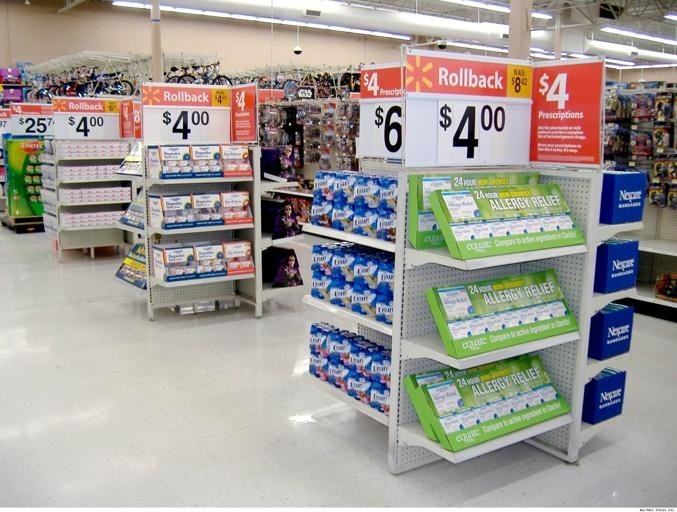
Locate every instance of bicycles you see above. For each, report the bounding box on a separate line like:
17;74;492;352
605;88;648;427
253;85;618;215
163;61;234;86
233;66;362;99
24;66;135;97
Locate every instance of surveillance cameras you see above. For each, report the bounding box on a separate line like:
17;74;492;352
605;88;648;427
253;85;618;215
437;39;447;50
293;45;303;56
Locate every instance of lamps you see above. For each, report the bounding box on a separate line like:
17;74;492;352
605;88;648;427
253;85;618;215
588;40;677;60
621;65;677;70
112;2;411;41
600;25;677;46
531;13;552;20
443;0;510;14
664;14;676;21
570;54;634;66
531;52;565;60
531;47;566;56
604;64;620;70
446;41;508;53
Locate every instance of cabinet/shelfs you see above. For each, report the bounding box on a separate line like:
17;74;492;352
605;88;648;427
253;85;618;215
0;133;53;234
601;87;677;309
37;140;143;264
258;103;360;226
299;158;644;476
112;141;304;321
